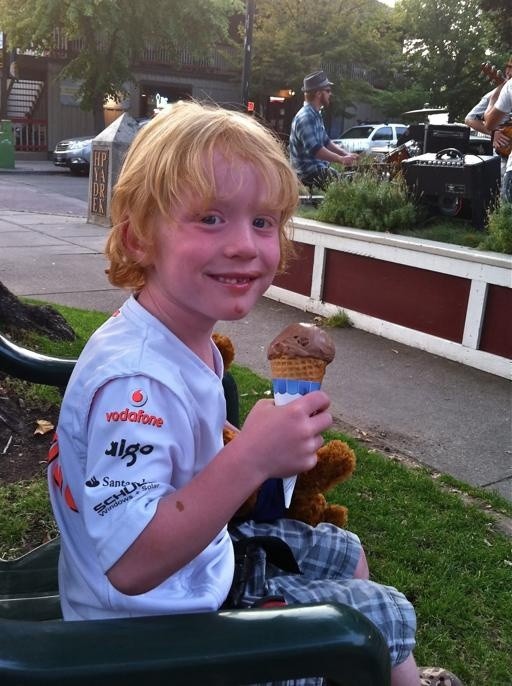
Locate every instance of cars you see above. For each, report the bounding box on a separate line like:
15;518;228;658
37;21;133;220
53;118;154;175
331;123;410;154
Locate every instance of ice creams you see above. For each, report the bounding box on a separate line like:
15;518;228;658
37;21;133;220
269;323;336;510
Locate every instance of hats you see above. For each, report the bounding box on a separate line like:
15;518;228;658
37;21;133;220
301;70;337;92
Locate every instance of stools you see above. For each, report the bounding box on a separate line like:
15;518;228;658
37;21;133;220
301;179;324;206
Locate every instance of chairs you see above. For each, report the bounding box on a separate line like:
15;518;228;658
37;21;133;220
0;332;392;685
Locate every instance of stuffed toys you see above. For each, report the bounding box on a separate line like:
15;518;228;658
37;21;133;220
210;333;357;530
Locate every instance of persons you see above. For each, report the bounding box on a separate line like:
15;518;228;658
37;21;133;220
464;64;512;186
46;98;461;686
485;76;512;205
290;70;357;193
